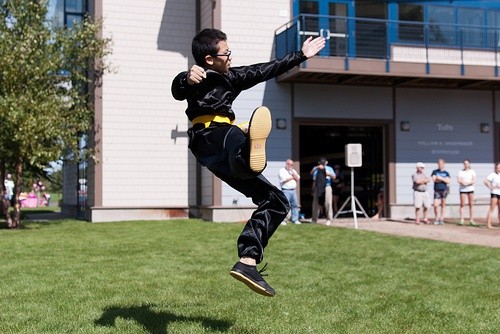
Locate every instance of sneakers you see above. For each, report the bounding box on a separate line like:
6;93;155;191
247;106;272;174
230;261;276;297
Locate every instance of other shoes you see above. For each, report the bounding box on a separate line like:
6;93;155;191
433;220;439;225
326;220;331;226
281;222;287;225
440;220;444;224
469;220;477;226
294;220;301;224
416;218;420;223
424;219;430;224
459;220;465;225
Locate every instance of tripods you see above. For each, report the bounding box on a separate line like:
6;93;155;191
333;166;374;230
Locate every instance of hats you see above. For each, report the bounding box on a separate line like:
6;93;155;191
416;162;424;167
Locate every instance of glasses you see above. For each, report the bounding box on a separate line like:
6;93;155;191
211;51;232;61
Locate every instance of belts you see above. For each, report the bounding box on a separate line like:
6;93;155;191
414;189;426;192
282;188;295;190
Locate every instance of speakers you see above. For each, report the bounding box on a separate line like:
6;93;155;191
345;144;363;167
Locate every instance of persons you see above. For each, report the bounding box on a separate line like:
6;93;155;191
331;164;343;214
0;173;51;230
278;159;301;225
457;160;476;226
411;162;431;225
311;157;337;226
483;162;500;228
171;28;326;296
430;159;451;226
